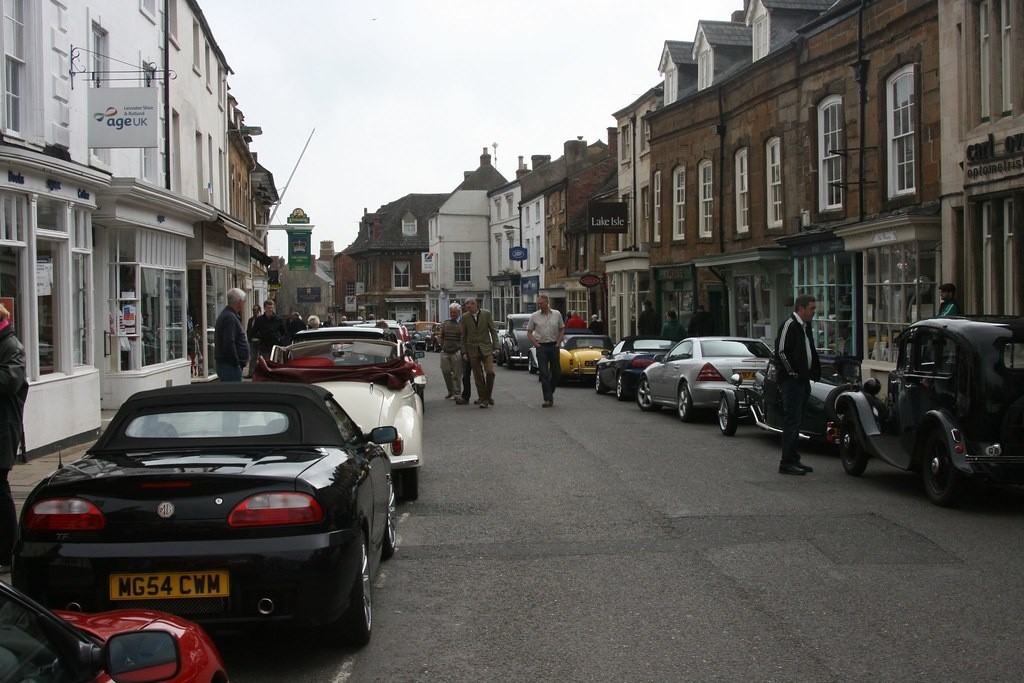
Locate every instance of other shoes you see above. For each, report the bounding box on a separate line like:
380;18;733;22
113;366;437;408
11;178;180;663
0;562;12;574
457;398;469;405
543;400;554;407
242;374;252;379
794;460;813;473
481;403;487;408
474;398;482;404
779;463;805;475
489;397;493;405
445;393;453;399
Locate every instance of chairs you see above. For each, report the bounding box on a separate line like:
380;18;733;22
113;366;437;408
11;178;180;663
139;422;177;438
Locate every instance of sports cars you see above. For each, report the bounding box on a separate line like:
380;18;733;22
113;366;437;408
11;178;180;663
10;380;402;648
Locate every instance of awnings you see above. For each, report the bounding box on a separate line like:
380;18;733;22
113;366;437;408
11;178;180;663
216;217;265;254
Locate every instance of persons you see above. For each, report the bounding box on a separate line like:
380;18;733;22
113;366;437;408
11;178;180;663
460;296;500;408
566;311;587;329
438;302;461;399
284;310;307;346
323;315;335;327
688;305;714;336
252;299;286;354
589;314;604;335
215;287;249;382
639;300;662;336
308;314;322;329
938;283;957;316
0;302;29;573
527;295;565;407
357;314;375;321
338;316;346;326
456;355;484;404
775;295;821;475
243;304;261;378
662;310;687;343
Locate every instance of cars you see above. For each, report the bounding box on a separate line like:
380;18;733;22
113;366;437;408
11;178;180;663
634;336;775;423
717;348;893;457
248;312;618;500
596;335;685;401
0;576;233;683
823;313;1024;507
